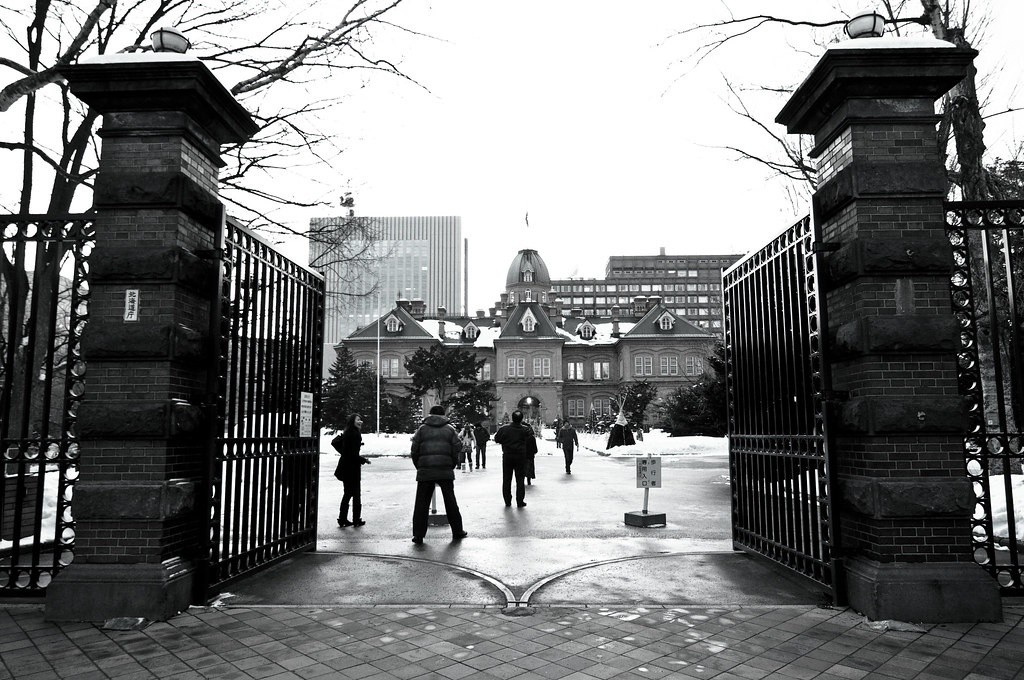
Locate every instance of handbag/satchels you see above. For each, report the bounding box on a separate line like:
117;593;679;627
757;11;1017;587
331;433;343;454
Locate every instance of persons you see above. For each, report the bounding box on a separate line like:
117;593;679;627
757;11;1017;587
411;405;467;543
494;410;542;507
334;414;371;527
556;414;579;474
458;423;490;472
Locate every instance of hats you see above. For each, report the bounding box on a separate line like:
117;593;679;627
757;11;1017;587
464;422;470;430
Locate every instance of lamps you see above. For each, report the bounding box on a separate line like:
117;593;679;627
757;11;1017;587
150;26;192;57
845;11;887;39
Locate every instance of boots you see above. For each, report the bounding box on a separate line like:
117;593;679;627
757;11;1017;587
469;462;473;472
461;463;466;473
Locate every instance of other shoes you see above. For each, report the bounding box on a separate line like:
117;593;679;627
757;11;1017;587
339;520;350;527
412;536;423;544
354;519;366;527
453;531;468;538
506;503;511;506
518;502;526;507
